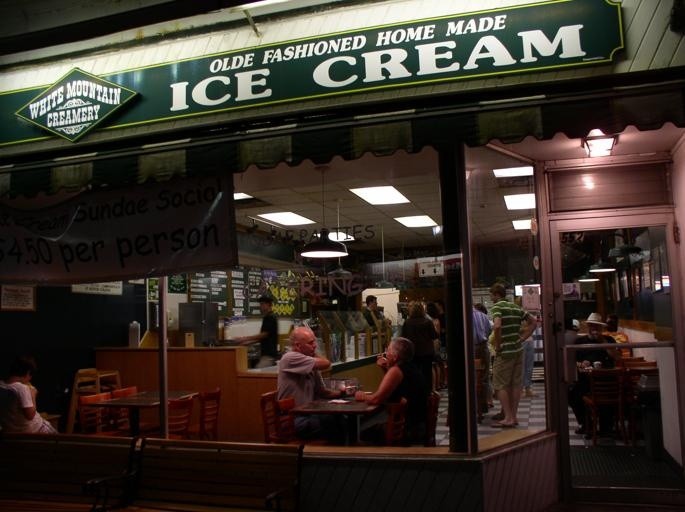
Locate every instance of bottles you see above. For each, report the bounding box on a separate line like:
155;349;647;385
128;321;140;347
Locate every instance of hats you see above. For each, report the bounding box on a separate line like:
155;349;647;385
572;320;580;330
581;313;608;328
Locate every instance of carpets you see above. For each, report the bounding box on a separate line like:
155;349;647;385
569;442;684;488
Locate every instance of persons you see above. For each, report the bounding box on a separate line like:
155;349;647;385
277;327;355;442
473;283;538;427
234;294;278;368
400;300;448;393
1;355;59;434
363;295;391;332
565;312;633;434
356;338;419;447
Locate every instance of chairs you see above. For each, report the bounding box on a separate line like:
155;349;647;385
576;356;663;458
65;367;441;449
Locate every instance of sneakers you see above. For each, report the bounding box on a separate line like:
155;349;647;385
526;388;539;397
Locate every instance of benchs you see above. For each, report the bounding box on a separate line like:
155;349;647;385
1;430;135;512
97;437;307;511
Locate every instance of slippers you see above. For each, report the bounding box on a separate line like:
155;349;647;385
491;412;518;428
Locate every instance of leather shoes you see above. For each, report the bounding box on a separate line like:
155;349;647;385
575;417;592;434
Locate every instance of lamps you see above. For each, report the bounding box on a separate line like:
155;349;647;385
298;166;349;259
579;127;620;159
327;199;353;280
373;220;396;289
